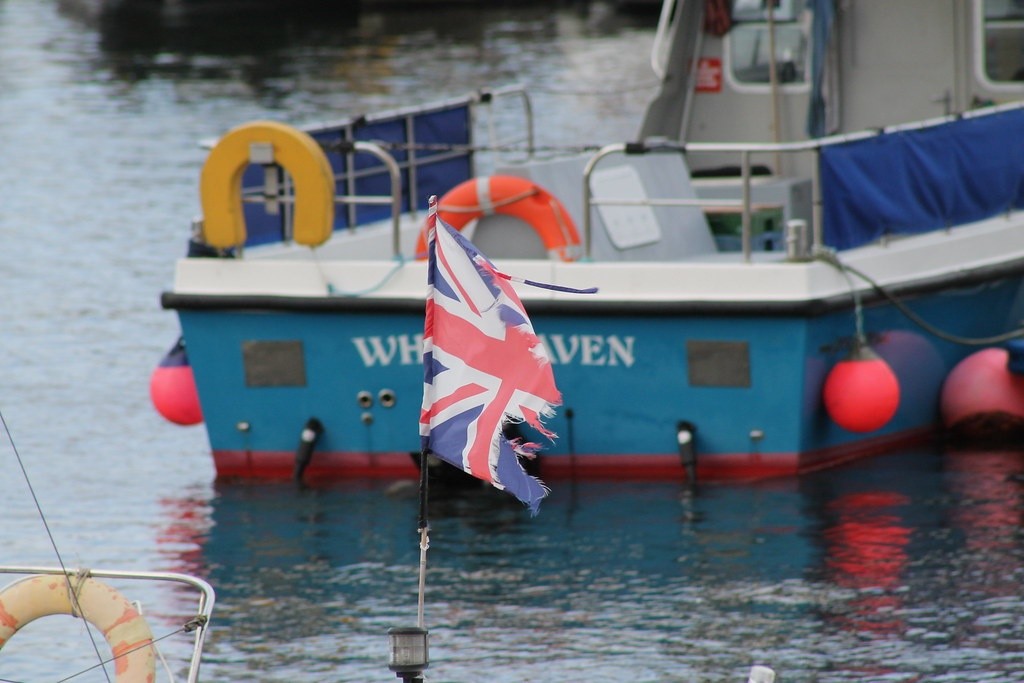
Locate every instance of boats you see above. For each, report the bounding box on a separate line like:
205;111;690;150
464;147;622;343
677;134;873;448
159;0;1024;484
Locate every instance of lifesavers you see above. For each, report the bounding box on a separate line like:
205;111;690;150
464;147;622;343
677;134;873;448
200;123;336;249
1;574;155;683
417;176;581;262
